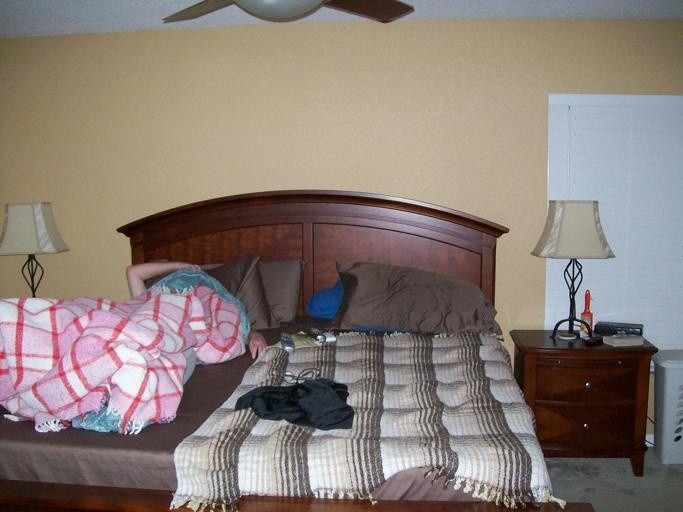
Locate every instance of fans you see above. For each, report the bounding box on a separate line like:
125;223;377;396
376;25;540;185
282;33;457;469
162;0;414;24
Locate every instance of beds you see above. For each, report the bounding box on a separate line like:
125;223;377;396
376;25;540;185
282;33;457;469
0;189;566;512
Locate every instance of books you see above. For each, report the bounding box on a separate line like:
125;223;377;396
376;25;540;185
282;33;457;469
594;321;643;336
602;334;644;347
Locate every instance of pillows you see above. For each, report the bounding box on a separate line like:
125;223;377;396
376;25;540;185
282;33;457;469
258;258;310;322
329;262;504;341
206;251;280;330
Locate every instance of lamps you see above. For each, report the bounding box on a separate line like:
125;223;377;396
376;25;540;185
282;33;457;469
233;0;322;22
531;200;616;337
0;203;70;298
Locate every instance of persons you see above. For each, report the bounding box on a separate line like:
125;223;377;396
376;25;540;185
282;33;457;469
127;261;268;360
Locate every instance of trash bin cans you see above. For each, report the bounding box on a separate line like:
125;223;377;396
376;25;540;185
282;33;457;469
650;350;683;465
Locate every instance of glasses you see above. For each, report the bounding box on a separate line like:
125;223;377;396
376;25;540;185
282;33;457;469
270;366;304;382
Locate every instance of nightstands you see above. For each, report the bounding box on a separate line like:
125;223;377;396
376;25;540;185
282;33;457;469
509;330;658;477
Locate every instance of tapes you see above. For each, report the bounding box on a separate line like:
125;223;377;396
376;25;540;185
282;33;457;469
557;332;578;340
